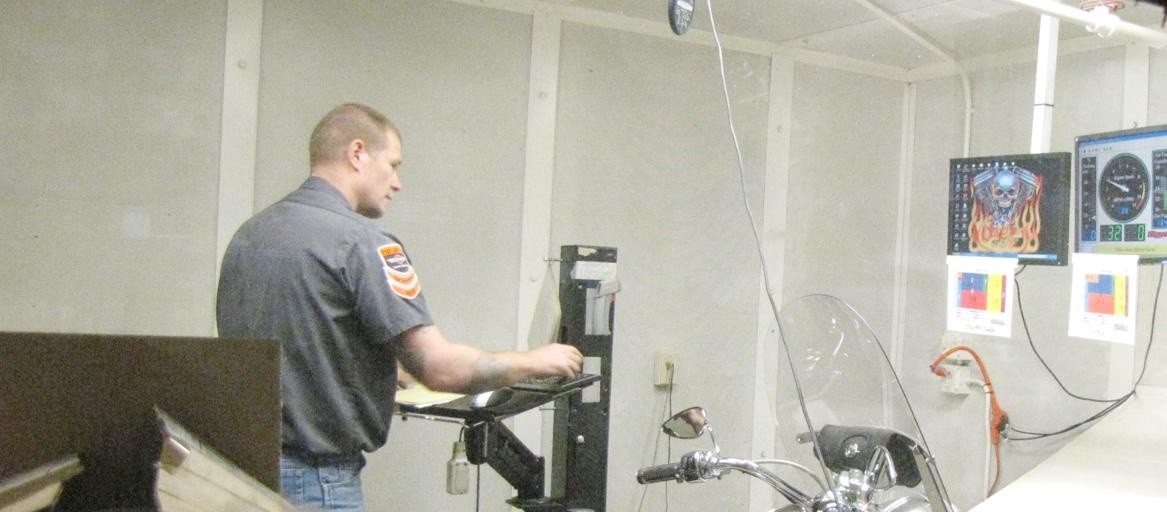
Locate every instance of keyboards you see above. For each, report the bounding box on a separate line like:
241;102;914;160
512;374;600;395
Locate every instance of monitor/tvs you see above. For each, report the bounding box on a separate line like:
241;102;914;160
945;151;1070;266
1075;127;1149;254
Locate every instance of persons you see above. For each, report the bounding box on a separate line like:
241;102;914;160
218;102;585;512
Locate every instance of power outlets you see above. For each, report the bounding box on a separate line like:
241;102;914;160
653;349;678;385
936;358;973;395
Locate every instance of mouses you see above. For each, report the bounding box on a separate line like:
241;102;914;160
469;385;510;408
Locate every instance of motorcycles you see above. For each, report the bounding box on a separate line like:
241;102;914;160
634;295;969;512
